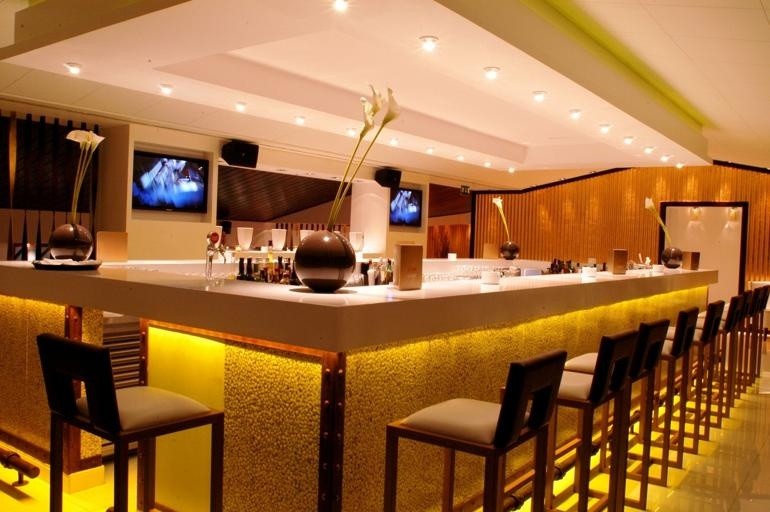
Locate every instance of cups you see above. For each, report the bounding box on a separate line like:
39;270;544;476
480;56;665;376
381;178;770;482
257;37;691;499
349;232;364;251
653;265;664;272
216;226;222;248
300;230;314;243
481;271;500;284
581;267;597;277
272;229;286;251
237;228;253;250
448;253;456;261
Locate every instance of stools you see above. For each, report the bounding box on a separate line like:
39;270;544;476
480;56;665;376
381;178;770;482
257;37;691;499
500;329;640;512
384;349;569;512
563;276;769;512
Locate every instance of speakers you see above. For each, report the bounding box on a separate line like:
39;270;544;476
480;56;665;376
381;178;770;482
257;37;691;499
375;168;401;188
222;139;259;168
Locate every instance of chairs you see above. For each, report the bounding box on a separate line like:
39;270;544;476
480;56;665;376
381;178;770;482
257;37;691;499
37;330;224;512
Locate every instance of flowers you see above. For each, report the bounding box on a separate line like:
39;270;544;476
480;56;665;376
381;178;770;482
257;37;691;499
491;197;512;242
326;85;402;232
643;197;675;247
64;128;106;226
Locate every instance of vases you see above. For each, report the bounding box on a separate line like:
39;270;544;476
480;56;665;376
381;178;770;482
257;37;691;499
500;241;520;260
660;246;682;270
49;221;95;262
291;227;356;295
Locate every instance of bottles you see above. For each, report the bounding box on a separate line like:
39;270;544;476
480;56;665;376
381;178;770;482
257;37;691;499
358;257;393;285
546;257;576;273
236;256;295;284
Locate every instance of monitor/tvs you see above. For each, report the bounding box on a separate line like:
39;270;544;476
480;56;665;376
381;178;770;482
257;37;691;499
389;187;422;229
132;146;210;216
